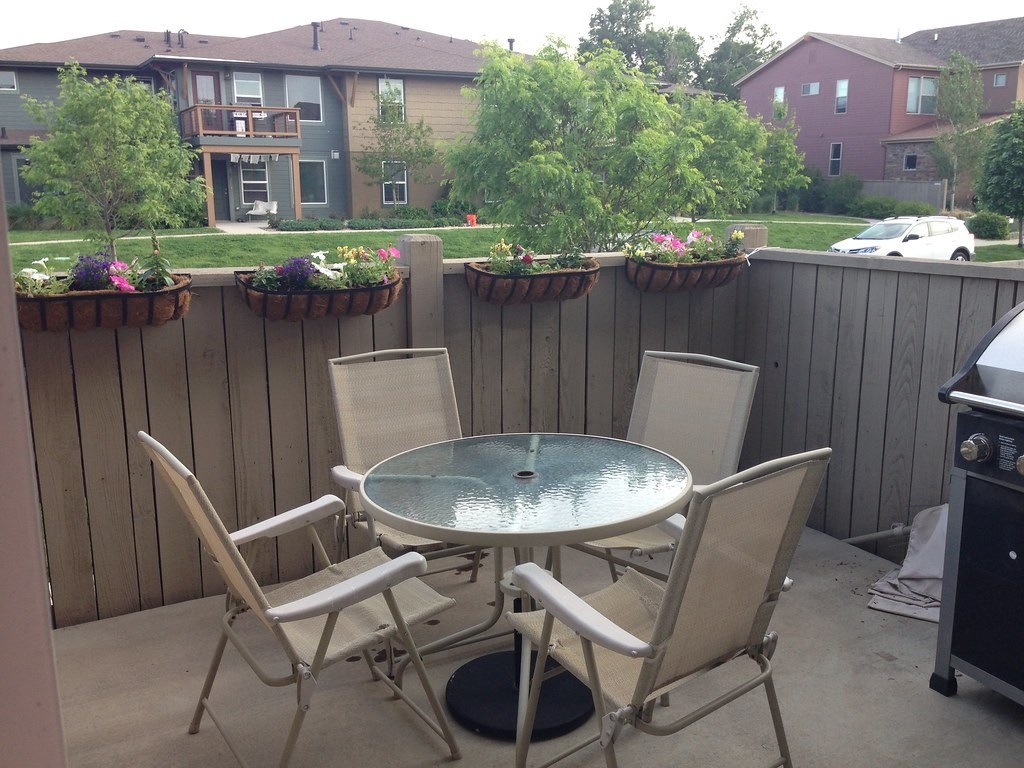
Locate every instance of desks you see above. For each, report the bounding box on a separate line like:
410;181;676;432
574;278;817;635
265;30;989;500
361;432;694;742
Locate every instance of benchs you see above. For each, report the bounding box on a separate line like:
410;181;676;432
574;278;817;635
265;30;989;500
245;199;278;222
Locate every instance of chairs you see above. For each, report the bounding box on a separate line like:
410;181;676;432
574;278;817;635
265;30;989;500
328;346;533;662
504;446;833;768
139;430;460;768
544;350;760;586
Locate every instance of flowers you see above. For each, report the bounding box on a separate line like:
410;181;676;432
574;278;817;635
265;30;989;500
623;226;745;265
488;229;587;274
12;226;174;296
250;243;401;293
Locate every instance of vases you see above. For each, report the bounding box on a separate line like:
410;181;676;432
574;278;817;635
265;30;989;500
625;251;746;293
14;275;190;332
235;270;401;323
463;256;600;304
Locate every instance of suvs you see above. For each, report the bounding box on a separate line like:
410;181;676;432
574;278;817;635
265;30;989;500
827;214;975;262
